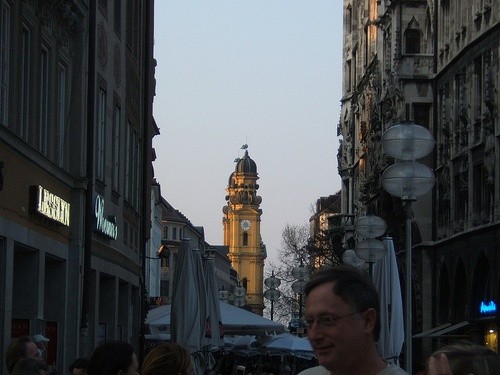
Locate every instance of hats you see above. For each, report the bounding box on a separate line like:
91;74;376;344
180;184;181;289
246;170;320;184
32;335;50;345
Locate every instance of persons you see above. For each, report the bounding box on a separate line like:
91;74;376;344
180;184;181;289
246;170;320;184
86;339;140;375
239;359;309;375
426;341;500;375
6;334;60;375
137;342;194;375
69;358;89;375
296;263;409;375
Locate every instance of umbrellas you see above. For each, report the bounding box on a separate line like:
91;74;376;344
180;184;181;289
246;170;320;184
372;233;404;368
169;238;225;375
220;332;317;360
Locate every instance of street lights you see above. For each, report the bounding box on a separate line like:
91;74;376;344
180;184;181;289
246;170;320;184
379;103;435;375
263;270;281;321
291;259;311;338
353;201;388;279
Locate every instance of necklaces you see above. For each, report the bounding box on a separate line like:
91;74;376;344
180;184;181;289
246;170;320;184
381;358;388;368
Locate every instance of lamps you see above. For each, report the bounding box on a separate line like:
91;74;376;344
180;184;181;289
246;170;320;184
141;245;170;261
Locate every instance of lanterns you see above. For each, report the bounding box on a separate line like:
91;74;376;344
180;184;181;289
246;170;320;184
264;289;281;301
380;161;436;201
292;265;310;281
355;214;387;239
265;276;281;289
379;120;436;162
354;238;387;263
341;249;366;267
234;286;246;296
291;279;307;295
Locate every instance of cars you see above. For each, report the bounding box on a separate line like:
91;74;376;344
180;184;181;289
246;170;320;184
288;318;307;334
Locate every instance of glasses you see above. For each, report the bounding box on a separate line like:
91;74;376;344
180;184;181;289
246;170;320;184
299;309;362;330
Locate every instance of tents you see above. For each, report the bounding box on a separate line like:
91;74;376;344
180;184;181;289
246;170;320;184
144;303;284;333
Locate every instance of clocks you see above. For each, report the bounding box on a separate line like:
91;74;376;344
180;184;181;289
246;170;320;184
241;219;252;230
